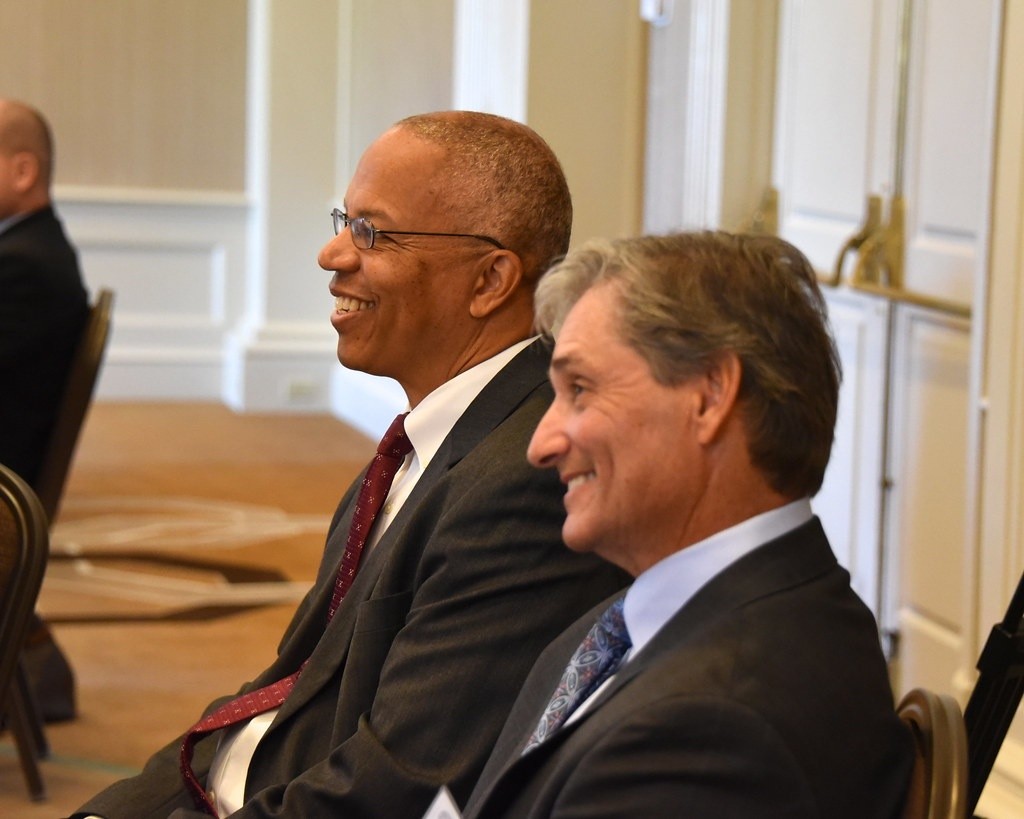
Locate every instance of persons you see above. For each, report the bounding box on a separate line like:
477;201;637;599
60;111;624;818
0;95;92;501
442;229;918;818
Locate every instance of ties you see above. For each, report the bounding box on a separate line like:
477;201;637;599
179;408;415;819
519;590;632;754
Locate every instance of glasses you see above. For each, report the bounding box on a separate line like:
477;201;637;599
330;207;506;252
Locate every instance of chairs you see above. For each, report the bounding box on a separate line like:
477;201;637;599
2;281;118;802
